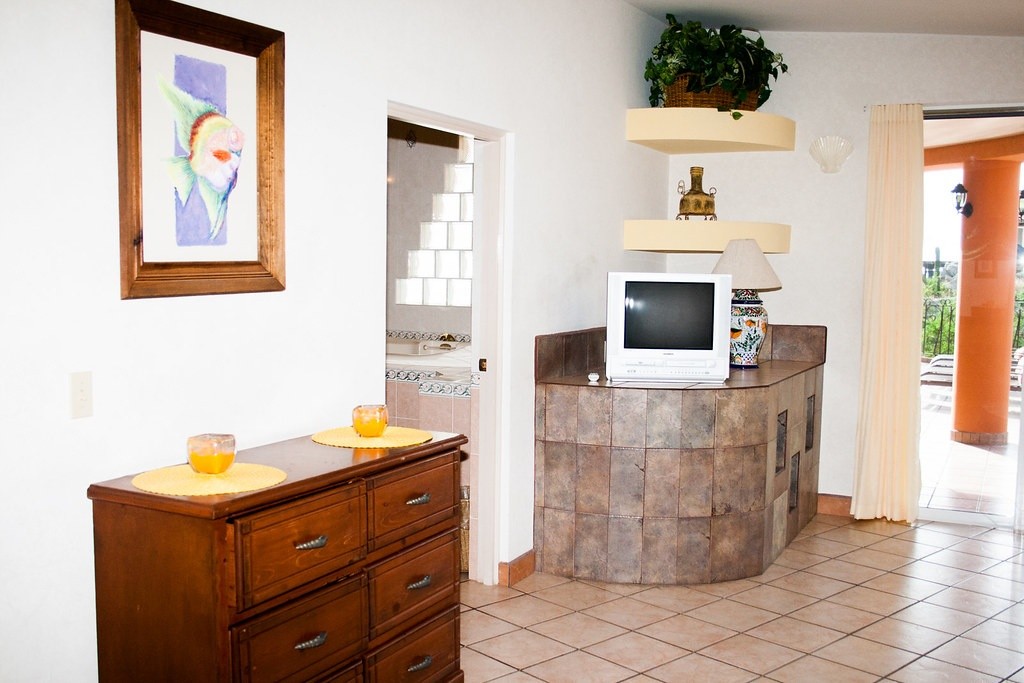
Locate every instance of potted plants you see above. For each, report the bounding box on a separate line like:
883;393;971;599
643;14;789;120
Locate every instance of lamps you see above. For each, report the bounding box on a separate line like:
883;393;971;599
1018;189;1024;229
951;184;973;218
713;239;783;370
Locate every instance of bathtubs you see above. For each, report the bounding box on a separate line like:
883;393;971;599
387;339;471;486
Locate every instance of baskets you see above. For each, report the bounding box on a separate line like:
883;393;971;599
662;72;758;112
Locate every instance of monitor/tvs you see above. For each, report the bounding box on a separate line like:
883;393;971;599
606;272;732;387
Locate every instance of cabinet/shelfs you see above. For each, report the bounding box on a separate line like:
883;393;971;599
626;106;796;254
87;425;465;683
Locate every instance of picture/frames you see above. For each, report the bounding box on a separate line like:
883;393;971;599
116;0;286;299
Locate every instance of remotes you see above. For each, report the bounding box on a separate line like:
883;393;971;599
588;373;599;381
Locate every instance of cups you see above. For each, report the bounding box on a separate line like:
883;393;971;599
188;434;236;474
354;405;389;438
